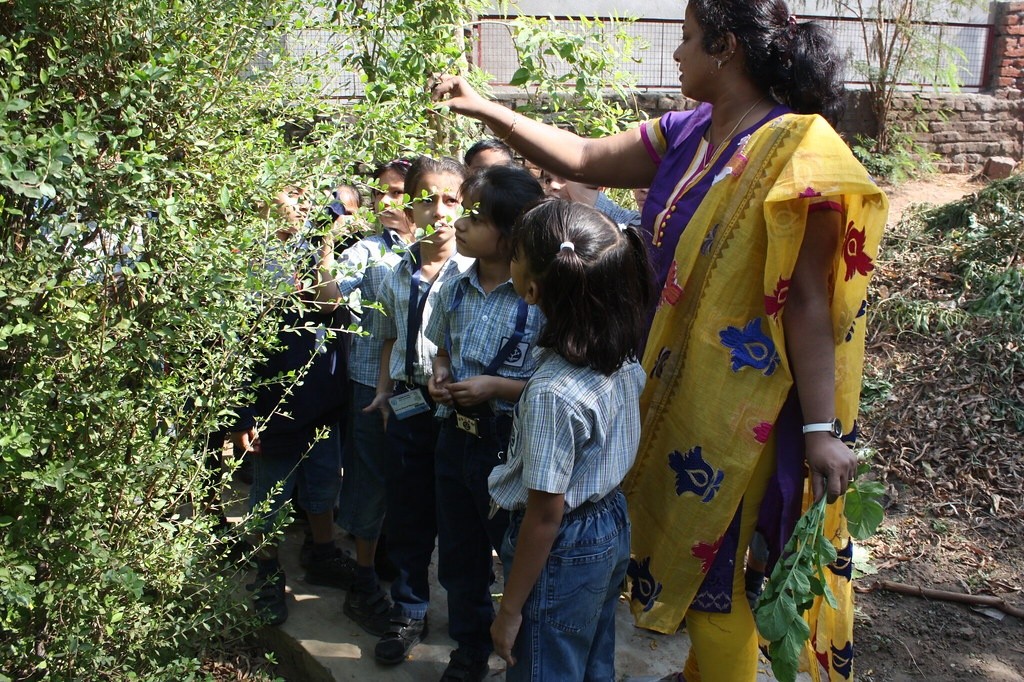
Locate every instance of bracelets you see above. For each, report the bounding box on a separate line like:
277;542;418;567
494;111;516;141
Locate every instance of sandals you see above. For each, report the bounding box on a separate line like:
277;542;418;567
375;614;428;664
343;582;396;635
440;648;489;682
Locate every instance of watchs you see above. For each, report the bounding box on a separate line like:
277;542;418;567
803;418;843;438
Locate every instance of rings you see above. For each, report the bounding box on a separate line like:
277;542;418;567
849;477;855;482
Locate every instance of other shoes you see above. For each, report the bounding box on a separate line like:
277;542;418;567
207;524;254;567
235;448;255;486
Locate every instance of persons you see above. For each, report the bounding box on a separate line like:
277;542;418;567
14;137;655;682
488;199;656;682
427;1;889;682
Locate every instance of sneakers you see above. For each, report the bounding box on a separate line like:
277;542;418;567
246;565;289;626
303;541;357;588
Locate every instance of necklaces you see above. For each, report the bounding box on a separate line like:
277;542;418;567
703;97;762;170
